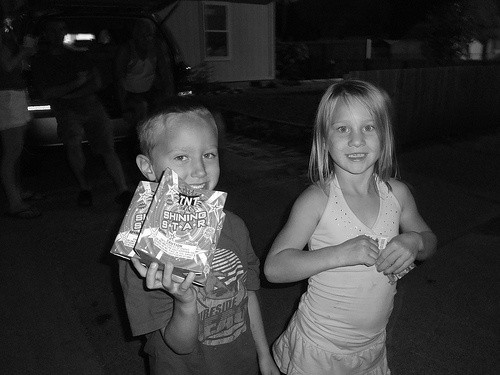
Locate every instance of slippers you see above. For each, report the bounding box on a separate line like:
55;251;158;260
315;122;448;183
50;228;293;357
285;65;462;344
7;189;46;218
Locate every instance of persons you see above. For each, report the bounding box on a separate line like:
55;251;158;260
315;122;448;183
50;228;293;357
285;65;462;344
117;17;170;123
0;18;47;218
367;40;403;70
262;78;437;375
33;20;134;207
108;98;282;375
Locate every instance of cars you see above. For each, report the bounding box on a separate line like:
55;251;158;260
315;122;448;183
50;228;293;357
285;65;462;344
18;0;195;169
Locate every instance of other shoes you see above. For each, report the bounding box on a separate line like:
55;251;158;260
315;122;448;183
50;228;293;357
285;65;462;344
80;189;93;207
119;190;132;201
